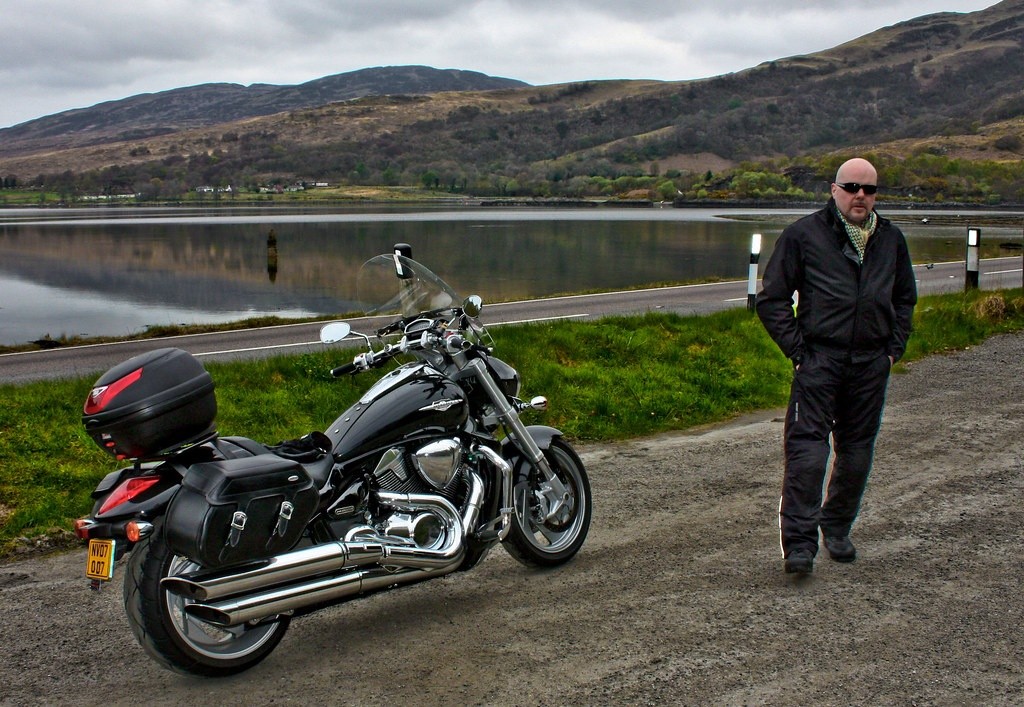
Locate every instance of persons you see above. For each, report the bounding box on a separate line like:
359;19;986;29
754;157;918;574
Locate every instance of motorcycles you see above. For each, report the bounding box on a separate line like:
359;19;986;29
75;252;590;679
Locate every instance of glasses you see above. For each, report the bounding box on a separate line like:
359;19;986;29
835;182;879;195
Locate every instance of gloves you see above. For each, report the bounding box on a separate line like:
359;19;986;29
262;431;333;463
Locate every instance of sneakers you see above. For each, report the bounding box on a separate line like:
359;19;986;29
822;532;857;563
785;549;815;574
